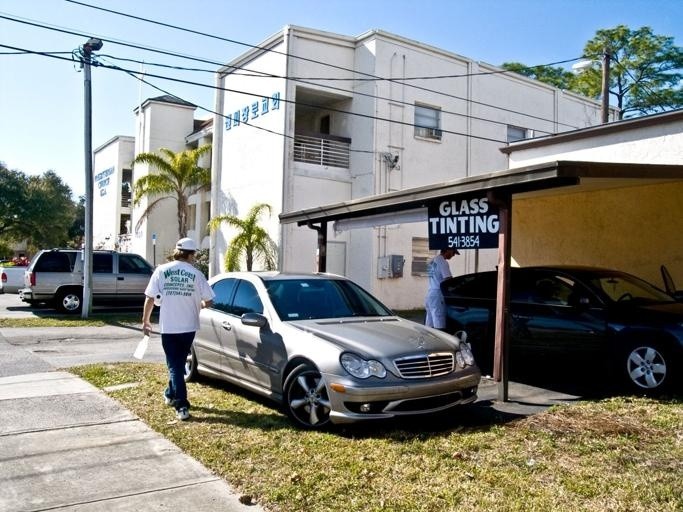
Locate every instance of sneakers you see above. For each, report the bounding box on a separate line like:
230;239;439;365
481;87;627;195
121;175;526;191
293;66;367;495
163;391;174;406
176;406;190;420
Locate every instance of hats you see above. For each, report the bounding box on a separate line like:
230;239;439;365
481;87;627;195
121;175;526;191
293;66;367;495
175;237;201;252
454;248;460;256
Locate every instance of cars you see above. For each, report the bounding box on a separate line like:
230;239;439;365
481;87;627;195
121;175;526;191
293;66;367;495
18;248;156;315
0;267;24;293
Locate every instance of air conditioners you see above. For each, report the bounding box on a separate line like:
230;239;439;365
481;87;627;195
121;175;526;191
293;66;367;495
428;127;443;138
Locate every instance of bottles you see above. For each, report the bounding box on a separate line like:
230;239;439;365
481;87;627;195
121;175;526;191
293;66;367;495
132;334;151;361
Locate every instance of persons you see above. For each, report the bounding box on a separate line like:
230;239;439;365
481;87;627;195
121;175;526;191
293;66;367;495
141;237;217;421
423;247;461;331
